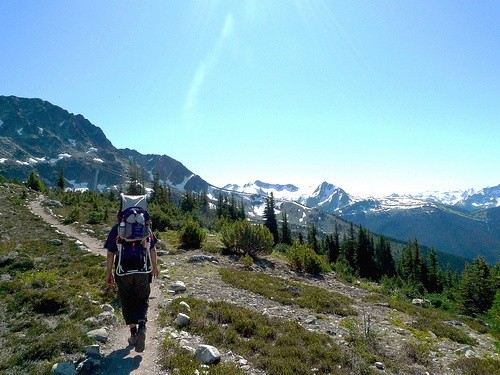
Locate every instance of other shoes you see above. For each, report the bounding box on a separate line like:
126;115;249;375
127;329;147;352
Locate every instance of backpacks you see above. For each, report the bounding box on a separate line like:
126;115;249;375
114;192;154;276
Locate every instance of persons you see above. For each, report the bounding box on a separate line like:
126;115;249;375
103;210;160;353
